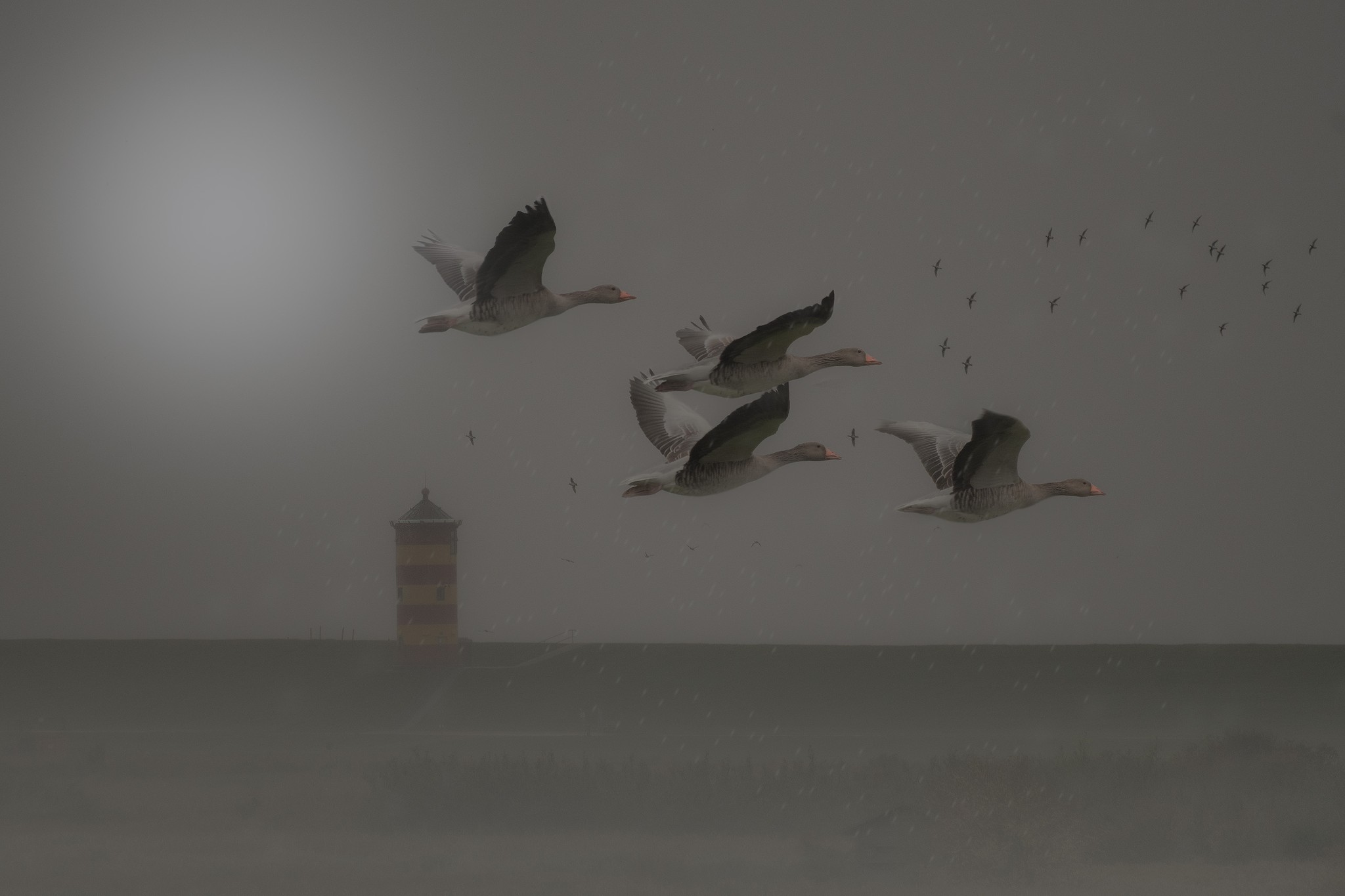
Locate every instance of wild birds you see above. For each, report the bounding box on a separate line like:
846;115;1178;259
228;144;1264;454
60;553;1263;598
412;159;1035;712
847;428;859;446
967;292;977;309
1262;279;1272;296
961;355;972;373
1208;236;1319;277
1179;284;1191;298
1219;322;1229;337
939;337;950;357
415;194;637;336
644;289;883;397
932;259;942;277
1144;210;1153;230
1045;227;1054;248
1191;215;1202;233
1079;228;1088;246
874;407;1107;521
1293;304;1302;322
462;429;766;561
620;368;842;496
1049;297;1061;314
